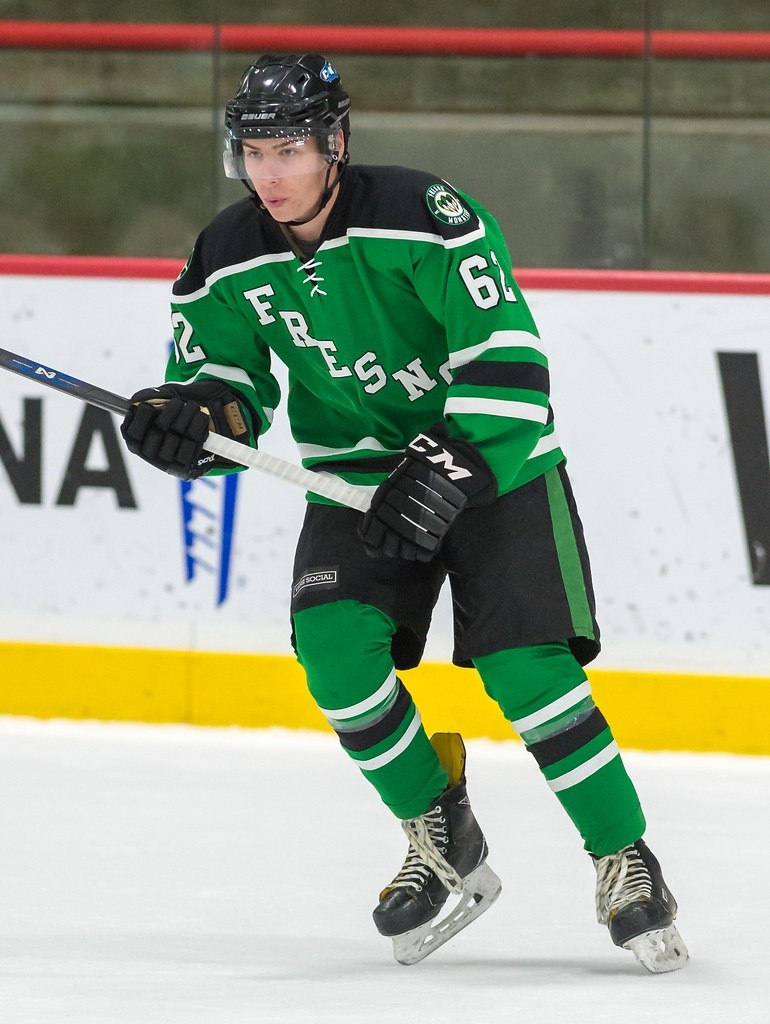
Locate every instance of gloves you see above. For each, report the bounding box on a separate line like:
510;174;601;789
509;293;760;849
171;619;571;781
120;384;249;480
359;424;497;567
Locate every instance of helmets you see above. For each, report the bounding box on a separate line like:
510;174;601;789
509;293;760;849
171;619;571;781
227;55;352;159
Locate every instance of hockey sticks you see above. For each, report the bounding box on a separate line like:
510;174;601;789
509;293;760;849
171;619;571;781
0;324;385;521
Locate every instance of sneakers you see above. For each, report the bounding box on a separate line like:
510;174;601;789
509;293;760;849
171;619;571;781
584;838;690;975
371;732;501;967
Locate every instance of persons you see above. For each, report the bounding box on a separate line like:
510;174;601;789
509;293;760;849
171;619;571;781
119;48;689;973
